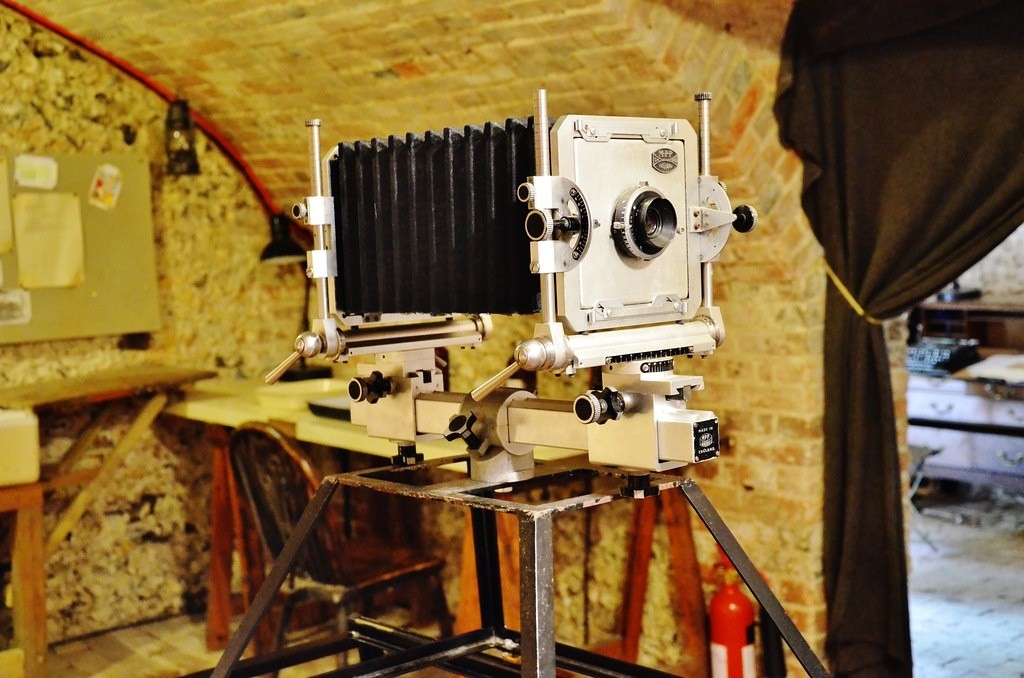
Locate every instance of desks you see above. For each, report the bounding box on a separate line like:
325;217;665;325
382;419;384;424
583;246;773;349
131;373;587;651
0;364;216;677
0;483;45;677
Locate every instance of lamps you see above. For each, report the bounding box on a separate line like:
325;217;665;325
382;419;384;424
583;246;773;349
161;100;203;175
257;213;332;383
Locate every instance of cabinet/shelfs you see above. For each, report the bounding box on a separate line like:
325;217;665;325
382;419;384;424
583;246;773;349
905;302;1024;494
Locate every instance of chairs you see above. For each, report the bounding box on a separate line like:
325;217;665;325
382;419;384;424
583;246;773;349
229;422;454;676
909;443;942;550
